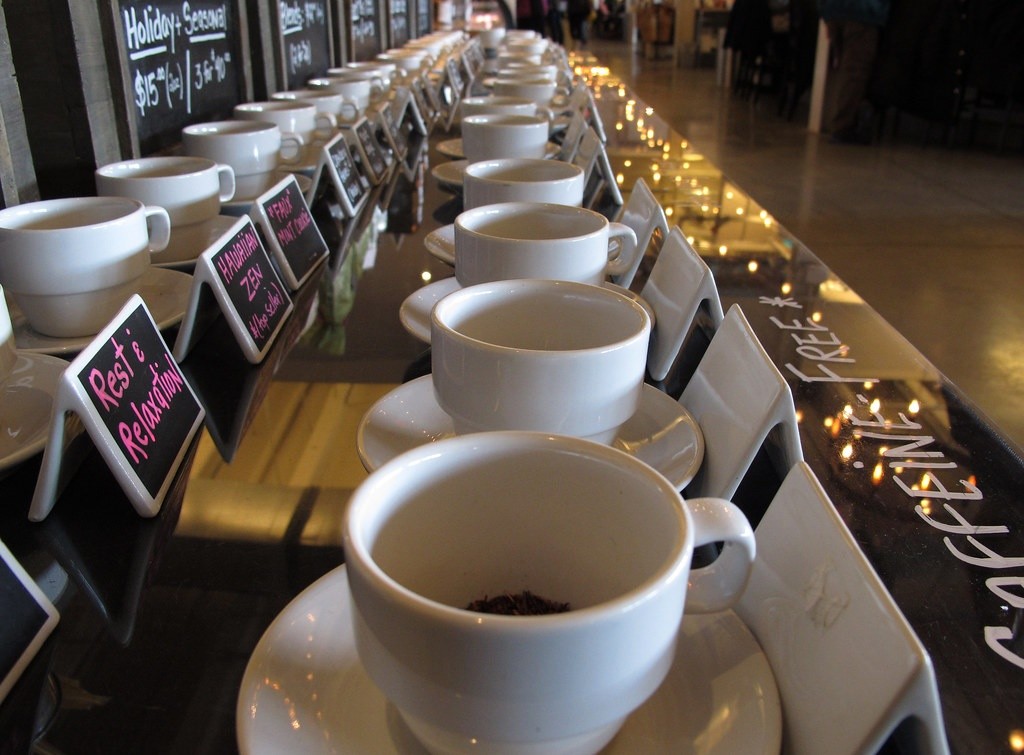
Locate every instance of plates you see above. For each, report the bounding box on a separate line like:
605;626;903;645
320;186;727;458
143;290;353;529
354;374;705;507
398;274;656;355
144;215;242;270
0;351;80;471
2;266;193;355
281;136;337;173
428;162;492;186
232;560;790;755
434;136;564;160
218;170;313;212
424;219;621;266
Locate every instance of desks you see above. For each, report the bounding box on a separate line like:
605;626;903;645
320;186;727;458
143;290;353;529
0;25;1024;755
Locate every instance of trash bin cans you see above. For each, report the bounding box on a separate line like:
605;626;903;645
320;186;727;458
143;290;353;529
717;27;741;89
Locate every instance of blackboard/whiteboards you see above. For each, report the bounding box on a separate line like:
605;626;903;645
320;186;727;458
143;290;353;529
110;0;254;161
389;0;432;49
277;0;334;90
349;0;386;62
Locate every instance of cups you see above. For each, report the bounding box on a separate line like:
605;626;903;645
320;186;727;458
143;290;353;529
343;25;756;755
0;23;476;474
